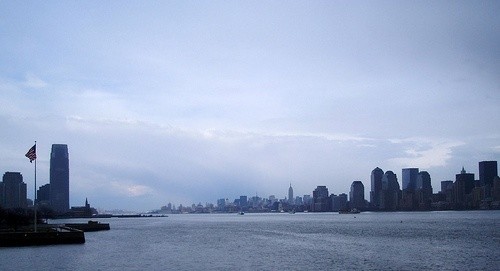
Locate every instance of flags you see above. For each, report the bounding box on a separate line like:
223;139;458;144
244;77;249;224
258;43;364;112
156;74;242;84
25;144;37;163
